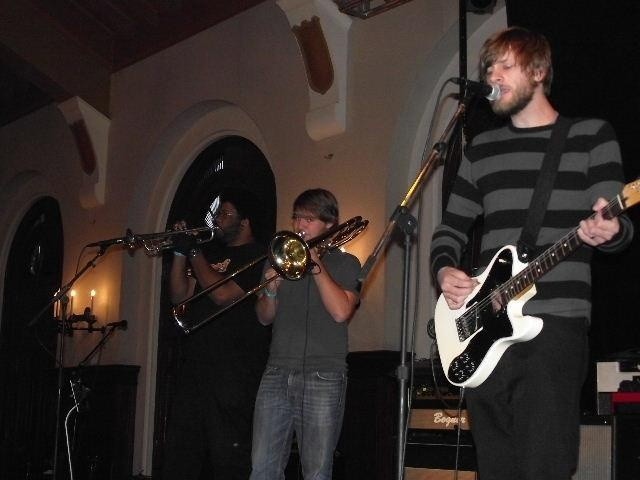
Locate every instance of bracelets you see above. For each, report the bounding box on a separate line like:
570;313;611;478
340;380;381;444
263;286;277;300
170;248;185;256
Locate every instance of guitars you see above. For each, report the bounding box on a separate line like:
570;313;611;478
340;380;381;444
433;178;639;391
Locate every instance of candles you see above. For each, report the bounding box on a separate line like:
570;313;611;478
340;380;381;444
90;296;94;314
70;296;73;317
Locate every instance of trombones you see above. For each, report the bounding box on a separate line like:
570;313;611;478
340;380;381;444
170;216;370;334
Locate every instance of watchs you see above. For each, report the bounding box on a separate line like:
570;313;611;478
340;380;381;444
190;249;200;256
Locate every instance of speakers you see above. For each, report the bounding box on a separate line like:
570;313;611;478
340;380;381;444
571;414;616;480
402;358;479;480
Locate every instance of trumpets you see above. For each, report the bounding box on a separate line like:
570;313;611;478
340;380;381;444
123;224;223;258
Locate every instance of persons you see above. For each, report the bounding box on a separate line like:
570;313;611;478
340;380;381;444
247;188;362;480
429;25;634;480
166;199;262;480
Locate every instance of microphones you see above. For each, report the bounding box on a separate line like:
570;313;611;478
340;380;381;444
107;320;128;327
451;76;503;102
87;236;131;247
